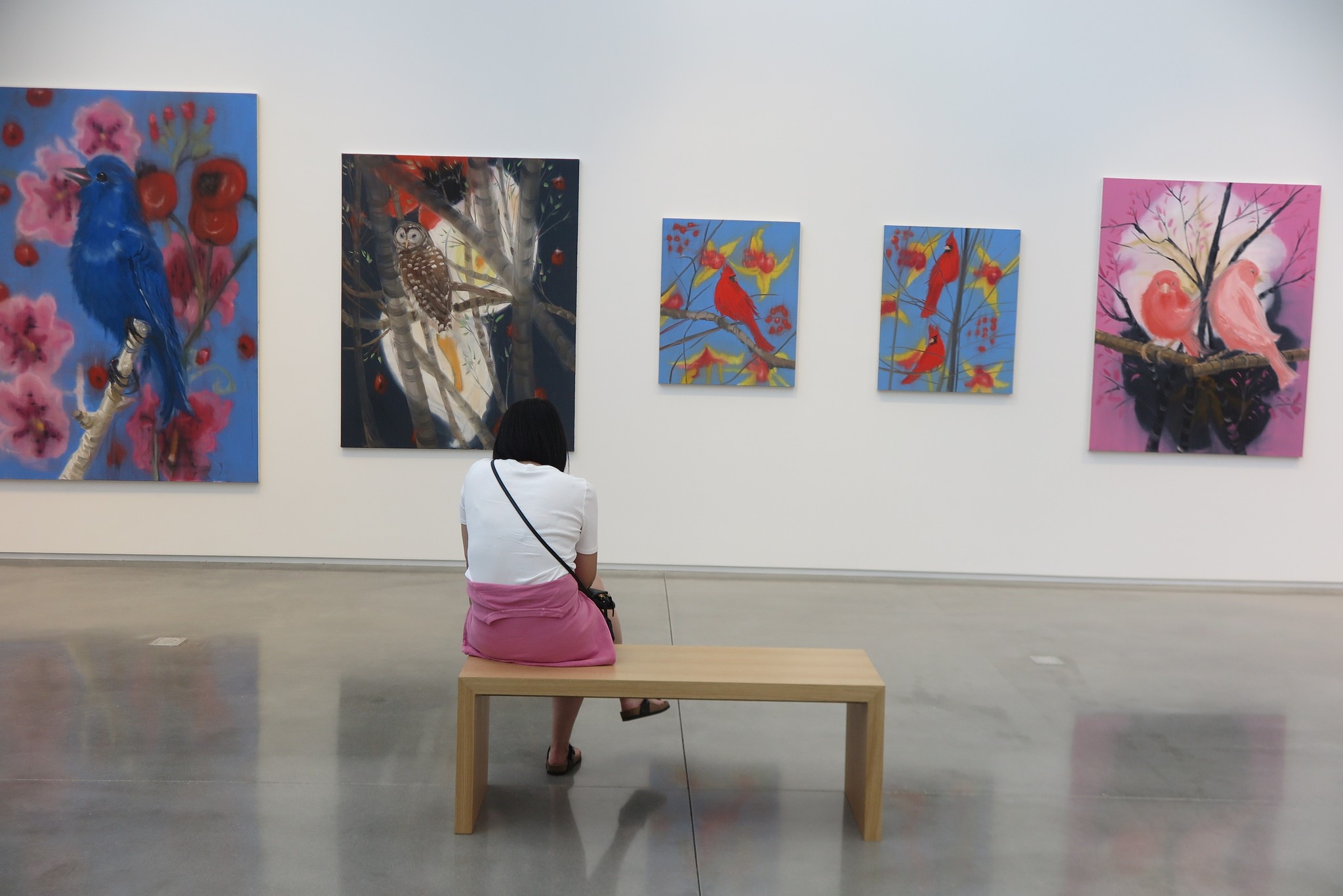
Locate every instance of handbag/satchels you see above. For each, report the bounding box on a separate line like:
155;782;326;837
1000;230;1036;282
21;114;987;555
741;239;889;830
588;586;616;642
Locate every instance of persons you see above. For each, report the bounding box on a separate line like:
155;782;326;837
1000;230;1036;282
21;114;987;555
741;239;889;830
458;398;671;775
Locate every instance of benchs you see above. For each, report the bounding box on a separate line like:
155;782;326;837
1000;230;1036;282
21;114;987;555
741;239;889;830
454;644;884;841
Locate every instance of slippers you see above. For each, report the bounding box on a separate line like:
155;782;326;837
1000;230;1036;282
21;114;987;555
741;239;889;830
620;697;670;721
545;742;582;775
